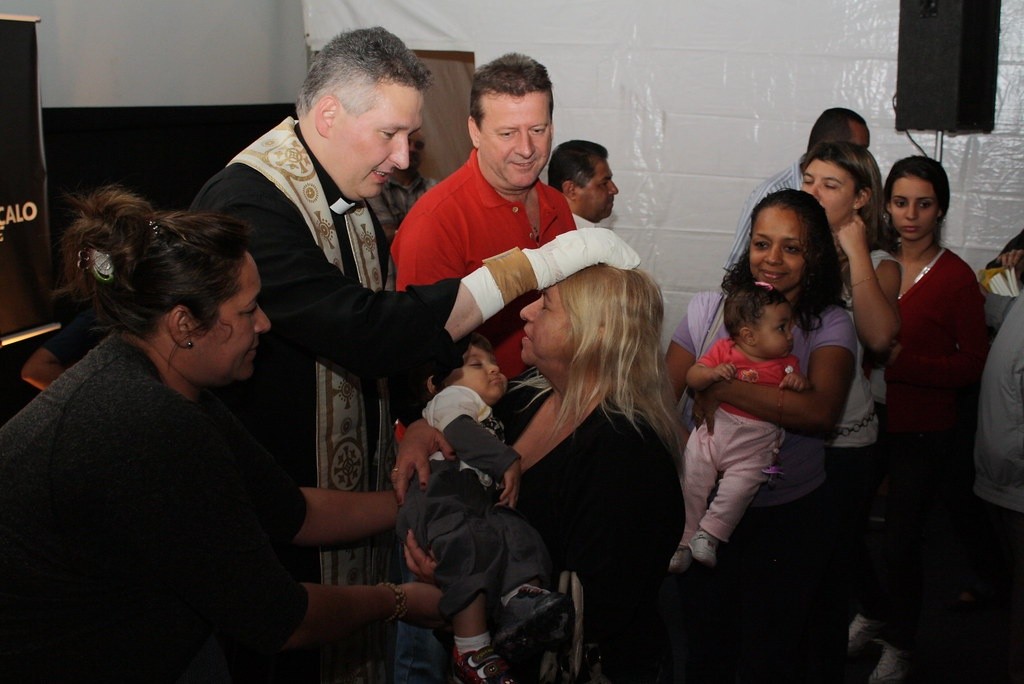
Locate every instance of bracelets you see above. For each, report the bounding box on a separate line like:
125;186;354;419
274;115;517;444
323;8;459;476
377;581;408;623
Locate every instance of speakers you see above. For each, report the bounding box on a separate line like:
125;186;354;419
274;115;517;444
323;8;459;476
895;0;1001;136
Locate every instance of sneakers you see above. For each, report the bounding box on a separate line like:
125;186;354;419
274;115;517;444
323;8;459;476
863;638;907;684
667;545;693;573
451;645;517;684
847;613;891;657
493;586;566;644
688;531;717;565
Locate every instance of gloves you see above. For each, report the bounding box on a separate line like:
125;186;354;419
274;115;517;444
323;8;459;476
482;227;641;308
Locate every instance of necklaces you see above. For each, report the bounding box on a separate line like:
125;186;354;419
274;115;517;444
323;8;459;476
530;222;540;242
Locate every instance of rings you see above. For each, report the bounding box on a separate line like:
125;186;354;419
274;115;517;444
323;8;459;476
392;467;400;472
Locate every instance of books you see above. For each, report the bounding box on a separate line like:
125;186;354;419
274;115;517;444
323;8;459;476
987;267;1019;297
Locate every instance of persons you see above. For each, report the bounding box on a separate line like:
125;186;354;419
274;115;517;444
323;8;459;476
960;225;1024;684
385;263;686;680
395;55;639;379
0;186;458;684
182;26;640;684
667;110;979;678
